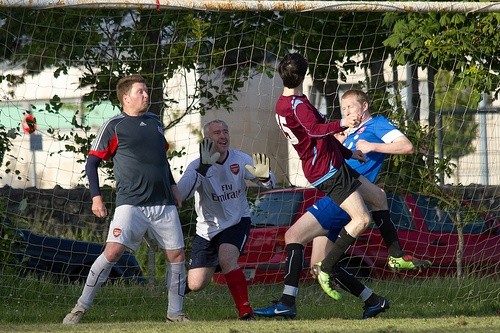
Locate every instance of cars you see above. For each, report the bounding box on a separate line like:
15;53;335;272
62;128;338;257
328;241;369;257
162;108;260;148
210;185;500;285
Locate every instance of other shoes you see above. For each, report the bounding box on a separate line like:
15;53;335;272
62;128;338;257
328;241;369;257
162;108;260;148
239;312;256;320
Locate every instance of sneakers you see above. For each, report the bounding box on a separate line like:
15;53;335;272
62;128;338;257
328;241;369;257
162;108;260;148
388;255;432;270
252;300;297;320
63;304;87;324
310;260;342;301
166;313;190;322
361;296;389;320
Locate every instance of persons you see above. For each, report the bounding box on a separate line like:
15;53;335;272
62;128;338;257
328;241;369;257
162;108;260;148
250;90;413;318
62;74;189;325
176;120;275;322
275;53;431;302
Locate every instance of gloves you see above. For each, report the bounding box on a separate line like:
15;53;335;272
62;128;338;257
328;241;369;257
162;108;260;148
195;138;220;177
245;153;271;183
